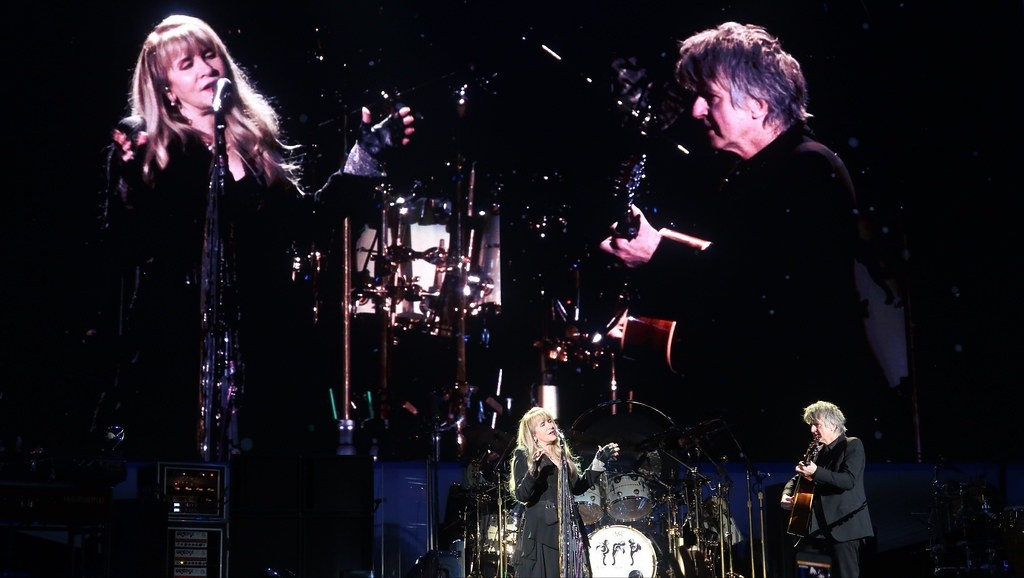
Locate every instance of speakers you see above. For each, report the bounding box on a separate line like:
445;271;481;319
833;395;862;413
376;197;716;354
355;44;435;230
228;453;375;578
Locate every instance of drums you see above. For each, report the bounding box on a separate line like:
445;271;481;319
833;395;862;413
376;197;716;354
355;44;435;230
349;207;503;329
477;513;520;562
570;482;604;527
588;525;657;578
604;472;653;523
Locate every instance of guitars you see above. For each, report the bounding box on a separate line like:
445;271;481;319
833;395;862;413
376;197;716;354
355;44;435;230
785;433;823;537
615;150;687;381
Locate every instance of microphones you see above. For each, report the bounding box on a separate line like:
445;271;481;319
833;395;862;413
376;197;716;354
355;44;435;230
560;441;565;460
211;77;232;111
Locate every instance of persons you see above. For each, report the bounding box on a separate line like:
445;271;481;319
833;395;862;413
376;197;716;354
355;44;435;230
509;406;620;578
108;13;415;477
599;22;865;532
781;401;874;577
